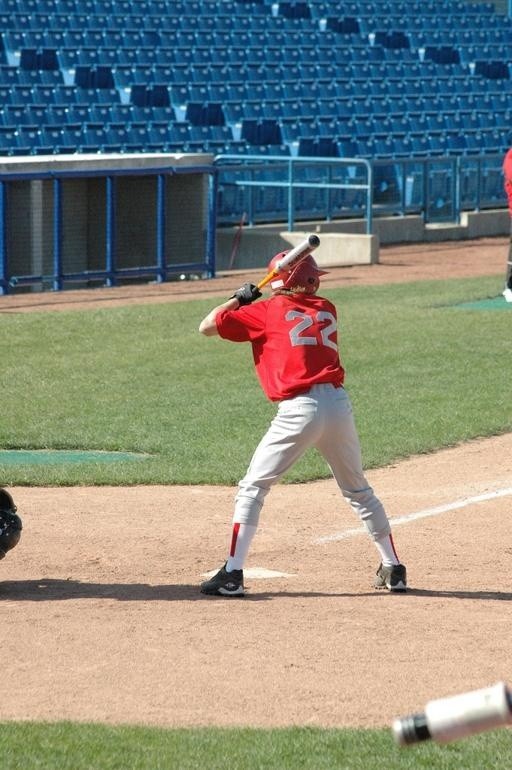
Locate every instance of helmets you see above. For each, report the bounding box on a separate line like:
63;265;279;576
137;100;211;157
267;248;330;294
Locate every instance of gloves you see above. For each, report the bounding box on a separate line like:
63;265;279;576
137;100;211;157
233;285;263;306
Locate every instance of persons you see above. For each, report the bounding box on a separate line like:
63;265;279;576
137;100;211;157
198;248;411;599
500;148;512;304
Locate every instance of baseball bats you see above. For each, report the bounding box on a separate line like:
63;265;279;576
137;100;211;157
252;236;320;292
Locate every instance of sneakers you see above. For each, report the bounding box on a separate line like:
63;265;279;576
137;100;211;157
368;562;409;593
198;564;247;596
